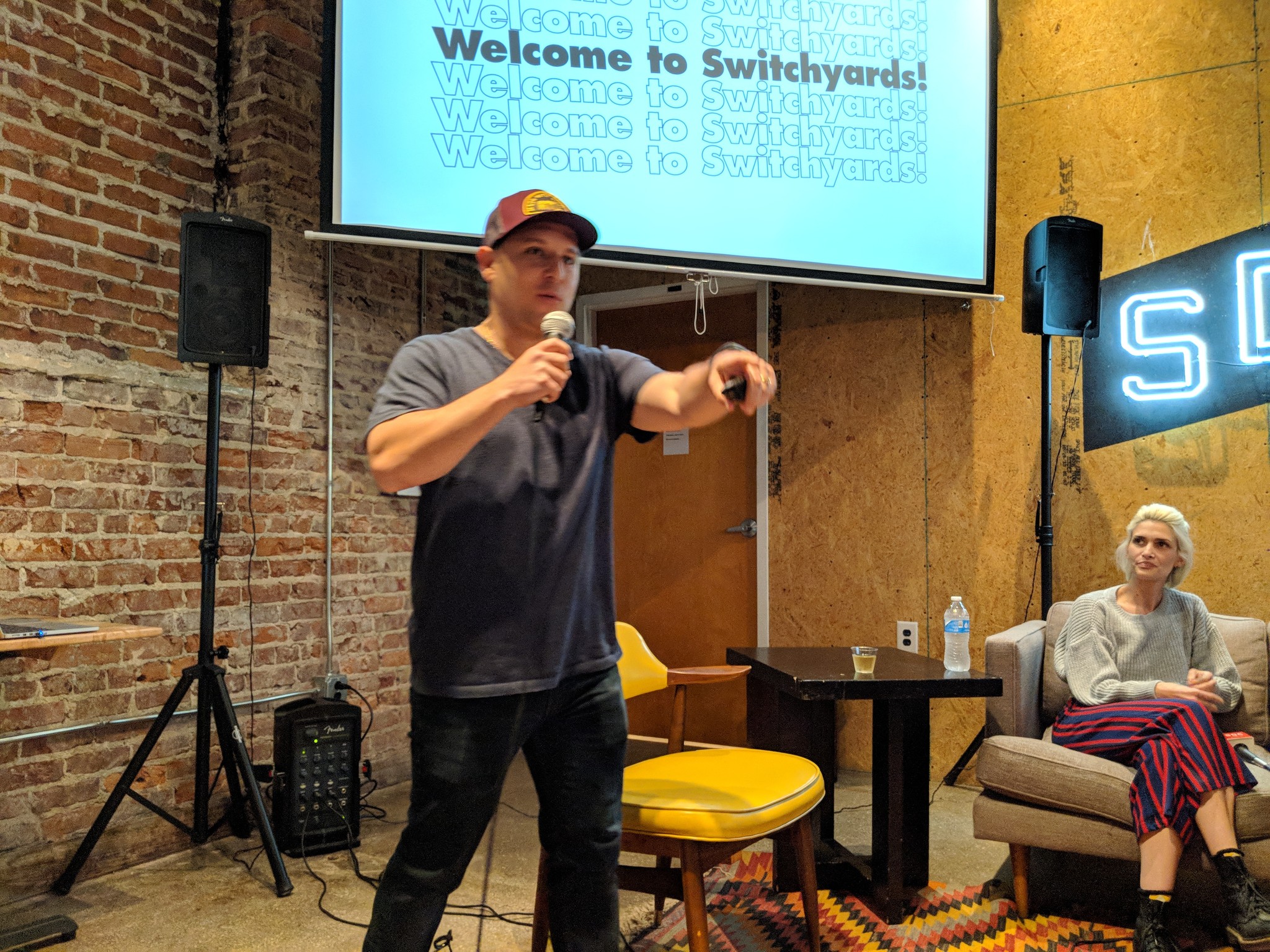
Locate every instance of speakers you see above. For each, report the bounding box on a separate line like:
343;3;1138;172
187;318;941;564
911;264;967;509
176;212;271;368
1021;216;1104;341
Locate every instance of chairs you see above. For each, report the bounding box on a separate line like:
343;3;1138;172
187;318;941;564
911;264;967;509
533;618;824;952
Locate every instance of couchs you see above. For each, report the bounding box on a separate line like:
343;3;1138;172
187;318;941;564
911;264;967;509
972;600;1270;922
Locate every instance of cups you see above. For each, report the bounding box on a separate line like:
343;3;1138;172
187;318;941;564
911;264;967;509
850;646;878;674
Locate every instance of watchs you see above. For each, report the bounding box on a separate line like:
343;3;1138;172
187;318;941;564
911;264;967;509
708;341;750;364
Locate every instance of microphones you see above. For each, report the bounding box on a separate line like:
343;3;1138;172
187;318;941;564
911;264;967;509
1223;731;1270;772
534;311;575;422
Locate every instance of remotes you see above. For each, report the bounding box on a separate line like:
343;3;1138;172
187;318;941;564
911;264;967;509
721;376;746;401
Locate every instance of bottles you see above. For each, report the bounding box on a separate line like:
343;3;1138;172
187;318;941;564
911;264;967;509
943;595;971;672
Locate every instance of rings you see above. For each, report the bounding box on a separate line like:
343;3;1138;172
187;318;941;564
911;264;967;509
760;375;772;385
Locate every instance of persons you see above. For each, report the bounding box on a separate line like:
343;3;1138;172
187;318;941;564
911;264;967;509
362;186;777;952
1052;503;1270;952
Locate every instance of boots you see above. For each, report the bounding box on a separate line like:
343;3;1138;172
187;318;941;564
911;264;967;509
1212;856;1270;952
1133;896;1172;952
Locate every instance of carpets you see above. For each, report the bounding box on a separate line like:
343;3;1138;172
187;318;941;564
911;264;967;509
618;850;1238;952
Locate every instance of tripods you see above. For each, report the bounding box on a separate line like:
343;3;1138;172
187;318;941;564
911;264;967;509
50;361;294;898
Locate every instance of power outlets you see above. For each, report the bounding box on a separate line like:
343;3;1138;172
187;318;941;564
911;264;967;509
896;620;918;654
314;673;349;701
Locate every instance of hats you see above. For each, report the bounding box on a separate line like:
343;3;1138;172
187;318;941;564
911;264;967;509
483;189;597;251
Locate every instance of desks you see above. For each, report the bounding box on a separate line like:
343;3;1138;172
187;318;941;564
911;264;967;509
0;613;162;952
727;644;1003;924
